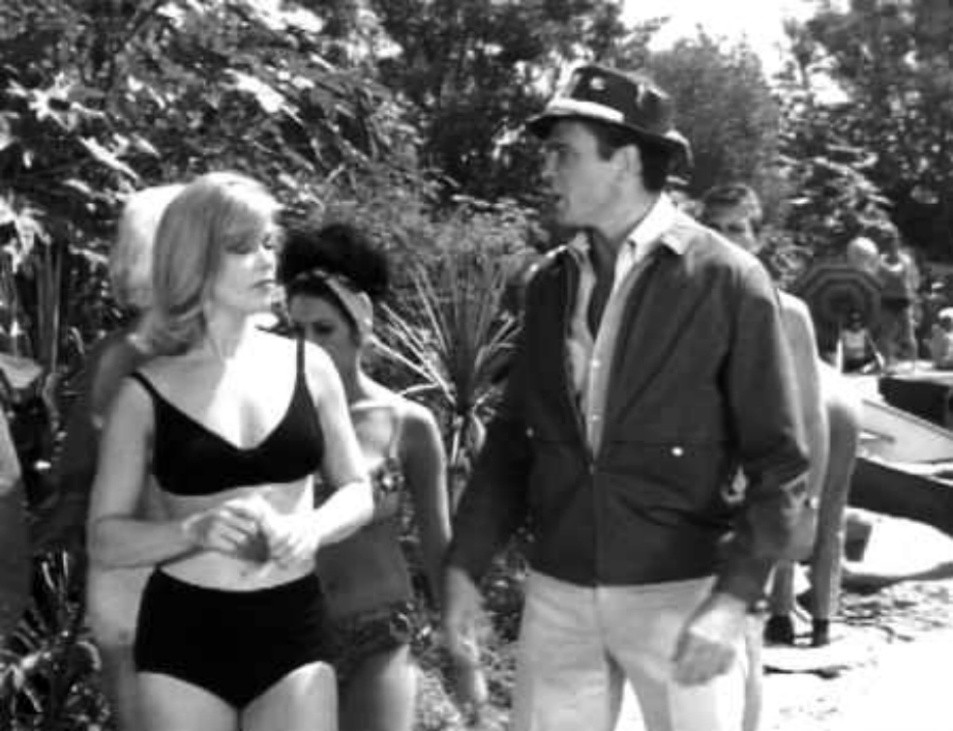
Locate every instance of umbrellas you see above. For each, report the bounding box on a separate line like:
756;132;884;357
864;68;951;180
787;259;887;362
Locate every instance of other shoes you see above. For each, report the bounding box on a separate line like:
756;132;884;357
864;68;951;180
808;617;830;647
762;615;794;646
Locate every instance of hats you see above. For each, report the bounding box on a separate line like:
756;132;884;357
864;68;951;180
521;64;687;152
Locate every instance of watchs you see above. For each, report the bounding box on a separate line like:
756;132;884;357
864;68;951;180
802;496;822;509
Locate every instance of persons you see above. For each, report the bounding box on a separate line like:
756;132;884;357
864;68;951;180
859;223;916;373
245;215;488;731
87;172;378;728
832;307;893;379
85;179;199;728
692;176;832;567
435;62;816;730
762;358;861;648
